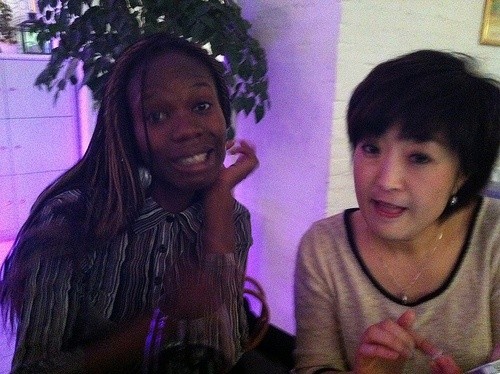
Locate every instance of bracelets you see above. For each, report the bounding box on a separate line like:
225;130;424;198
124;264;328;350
150;302;180;369
141;307;158;373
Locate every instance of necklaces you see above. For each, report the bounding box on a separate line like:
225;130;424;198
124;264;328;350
367;201;450;307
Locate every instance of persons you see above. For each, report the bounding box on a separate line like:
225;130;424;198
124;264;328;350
295;45;500;373
2;31;296;374
